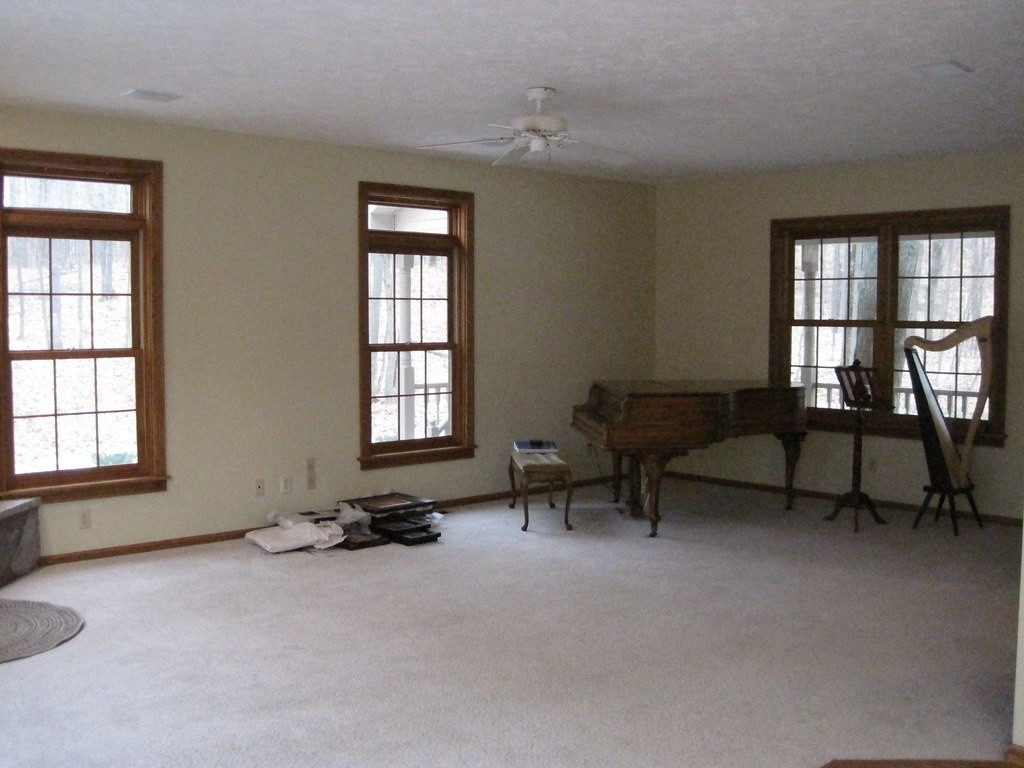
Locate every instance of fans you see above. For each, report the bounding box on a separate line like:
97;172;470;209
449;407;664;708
417;88;629;166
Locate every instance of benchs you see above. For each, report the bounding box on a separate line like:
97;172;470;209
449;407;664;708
509;451;574;529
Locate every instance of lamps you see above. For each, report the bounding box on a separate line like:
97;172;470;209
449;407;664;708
529;137;548;152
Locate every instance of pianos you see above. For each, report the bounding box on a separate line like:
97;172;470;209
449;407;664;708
569;378;810;538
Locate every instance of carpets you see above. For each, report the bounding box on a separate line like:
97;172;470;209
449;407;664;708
0;598;84;662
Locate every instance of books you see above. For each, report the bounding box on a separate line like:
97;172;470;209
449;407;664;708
513;440;558;454
